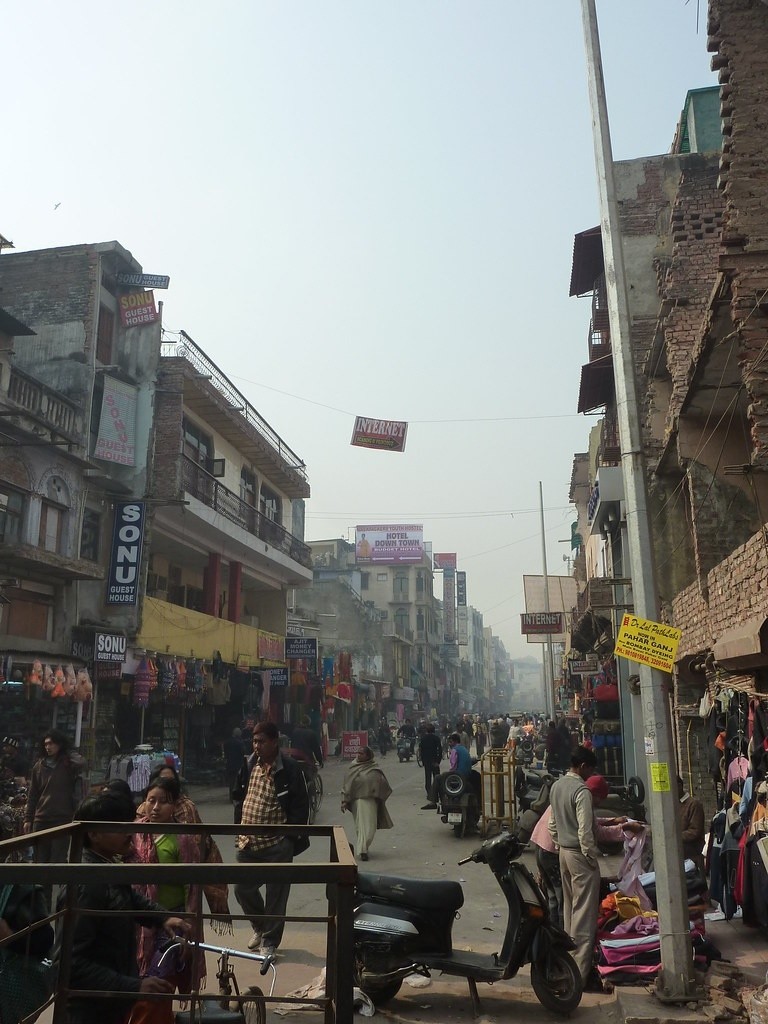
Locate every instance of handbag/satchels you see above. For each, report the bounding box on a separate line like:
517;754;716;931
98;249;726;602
591;720;620;735
597;702;620;720
592;683;619;704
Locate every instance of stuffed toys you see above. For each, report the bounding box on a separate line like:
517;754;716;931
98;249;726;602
29;660;93;701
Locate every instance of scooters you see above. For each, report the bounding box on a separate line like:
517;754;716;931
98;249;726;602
436;756;486;839
515;734;534;768
395;733;417;763
350;833;585;1021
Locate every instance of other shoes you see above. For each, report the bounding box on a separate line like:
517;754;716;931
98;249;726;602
380;753;386;759
361;853;368;861
477;756;485;761
427;796;439;802
421;804;437;809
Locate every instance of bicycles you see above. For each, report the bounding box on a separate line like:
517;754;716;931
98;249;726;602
416;731;446;767
307;762;325;825
156;935;276;1023
445;732;453;759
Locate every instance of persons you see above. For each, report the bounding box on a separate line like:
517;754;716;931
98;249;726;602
379;712;572;769
530;775;644;932
550;746;600;991
1;728;226;1024
642;775;705;873
340;746;392;861
291;715;323;800
231;721;309;965
421;734;471;810
419;725;442;801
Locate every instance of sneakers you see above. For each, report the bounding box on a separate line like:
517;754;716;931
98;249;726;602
247;933;276;965
